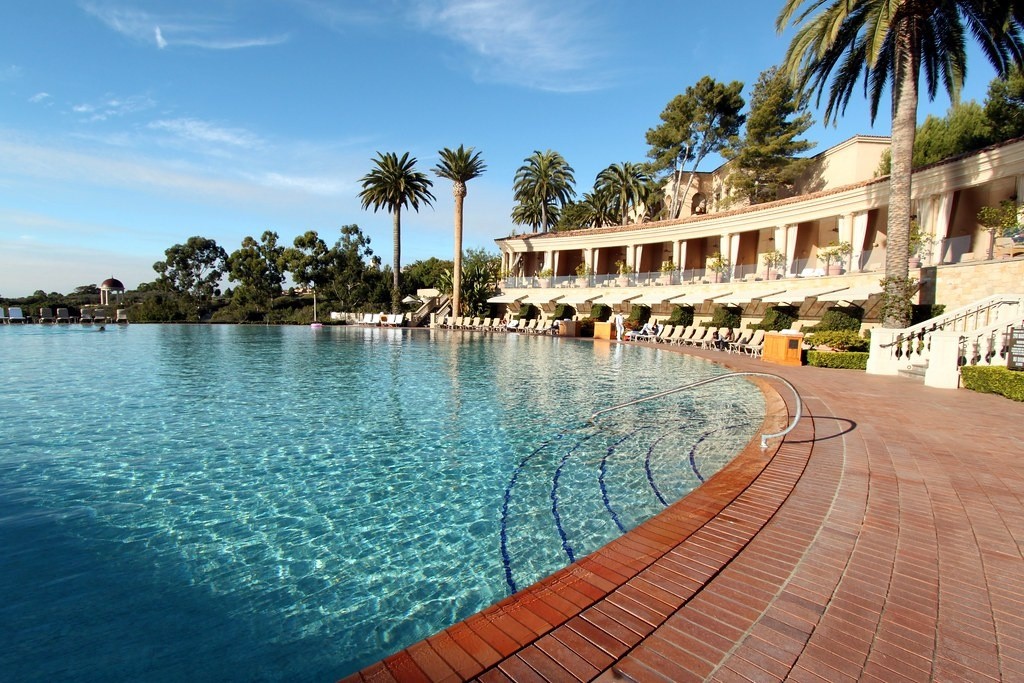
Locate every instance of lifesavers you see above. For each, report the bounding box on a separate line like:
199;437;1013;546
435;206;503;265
310;323;322;328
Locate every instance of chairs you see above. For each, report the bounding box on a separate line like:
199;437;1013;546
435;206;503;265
624;320;815;361
330;312;405;327
116;309;129;324
94;309;106;322
994;235;1024;258
8;307;26;324
436;316;564;336
39;307;55;325
0;307;8;324
79;308;93;322
554;267;825;288
56;308;71;324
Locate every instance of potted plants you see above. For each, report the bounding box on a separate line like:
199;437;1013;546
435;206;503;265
657;260;677;285
816;240;851;275
496;268;514;288
706;252;729;283
538;268;553;288
907;220;925;269
575;262;593;288
763;250;786;281
614;261;634;287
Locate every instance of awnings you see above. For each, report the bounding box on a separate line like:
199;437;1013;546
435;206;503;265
487;286;918;303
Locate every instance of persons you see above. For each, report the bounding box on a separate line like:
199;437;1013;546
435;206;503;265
721;327;735;350
615;310;624;341
639;325;657;335
713;332;725;351
548;322;559;336
